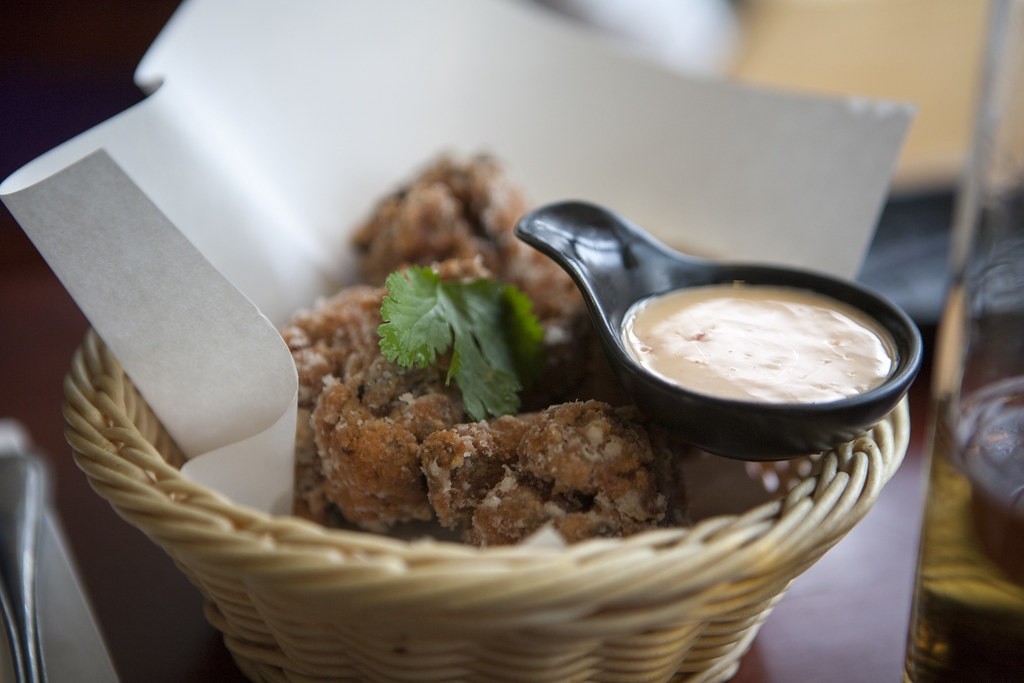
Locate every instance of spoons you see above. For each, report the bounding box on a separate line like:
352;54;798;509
514;200;922;461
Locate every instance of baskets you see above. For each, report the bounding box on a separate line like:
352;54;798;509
64;325;910;683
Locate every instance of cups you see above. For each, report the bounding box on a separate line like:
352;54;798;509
952;0;1024;586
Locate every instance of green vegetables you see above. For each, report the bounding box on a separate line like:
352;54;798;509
378;271;546;418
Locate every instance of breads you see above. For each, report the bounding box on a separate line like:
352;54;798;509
285;148;681;542
